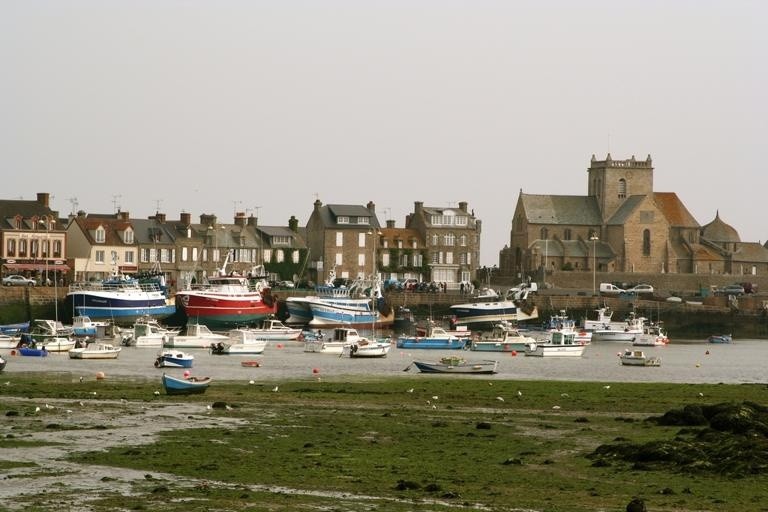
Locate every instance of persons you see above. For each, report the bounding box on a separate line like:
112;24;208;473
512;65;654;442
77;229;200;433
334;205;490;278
465;283;469;292
460;282;464;293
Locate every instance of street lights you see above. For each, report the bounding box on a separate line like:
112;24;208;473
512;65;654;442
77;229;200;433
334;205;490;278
367;226;382;340
590;231;598;296
38;214;56;287
207;216;225;276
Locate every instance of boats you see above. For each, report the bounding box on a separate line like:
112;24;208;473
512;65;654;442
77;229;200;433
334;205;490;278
161;373;212;395
176;271;281;329
709;333;732;344
285;286;394;332
67;273;175;326
395;299;671;380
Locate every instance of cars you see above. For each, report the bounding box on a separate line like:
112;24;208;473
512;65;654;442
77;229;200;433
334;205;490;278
627;284;654;296
2;274;37;287
714;284;744;297
599;283;626;296
510;283;537;296
